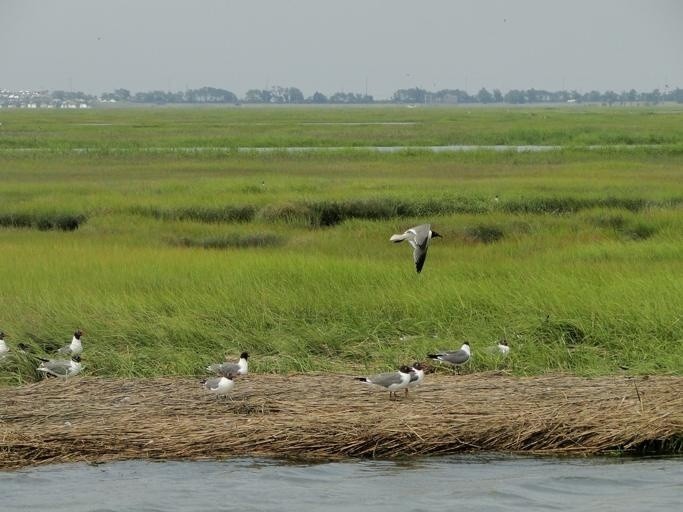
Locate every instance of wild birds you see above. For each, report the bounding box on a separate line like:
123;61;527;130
34;330;83;382
353;361;425;397
200;372;238;400
205;352;250;377
0;332;29;359
487;339;510;357
426;341;471;375
389;223;443;274
